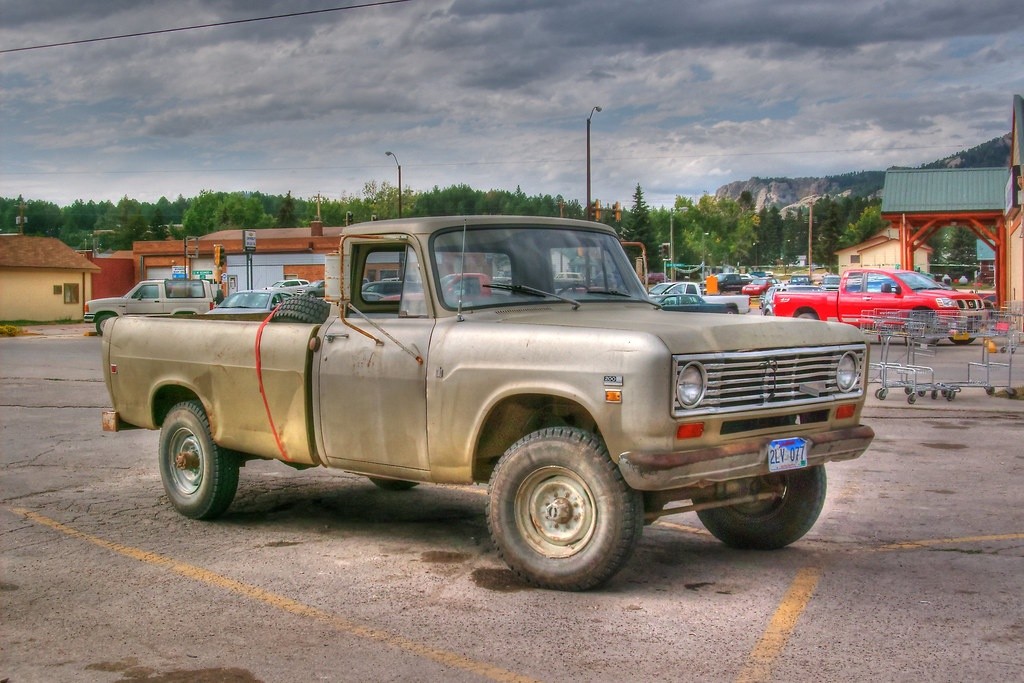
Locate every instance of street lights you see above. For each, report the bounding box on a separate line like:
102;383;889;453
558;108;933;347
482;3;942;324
385;151;402;219
587;107;602;217
701;232;709;291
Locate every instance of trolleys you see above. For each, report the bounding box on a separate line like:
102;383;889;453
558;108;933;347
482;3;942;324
858;307;1024;404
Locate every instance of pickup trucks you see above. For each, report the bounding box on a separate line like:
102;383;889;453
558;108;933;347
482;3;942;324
648;281;752;314
774;268;993;343
104;215;876;590
649;294;727;313
701;272;753;292
380;272;494;303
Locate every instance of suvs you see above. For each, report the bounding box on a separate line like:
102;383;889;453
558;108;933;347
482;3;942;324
648;272;664;283
553;272;585;286
820;274;843;291
742;277;778;295
760;284;826;316
591;271;622;288
83;280;215;335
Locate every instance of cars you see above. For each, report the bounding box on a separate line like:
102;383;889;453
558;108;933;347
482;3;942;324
361;277;420;302
204;279;325;313
789;273;814;284
741;271;773;279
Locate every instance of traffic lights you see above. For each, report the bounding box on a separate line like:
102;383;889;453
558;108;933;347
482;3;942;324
658;244;670;259
592;199;600;220
612;202;621;222
